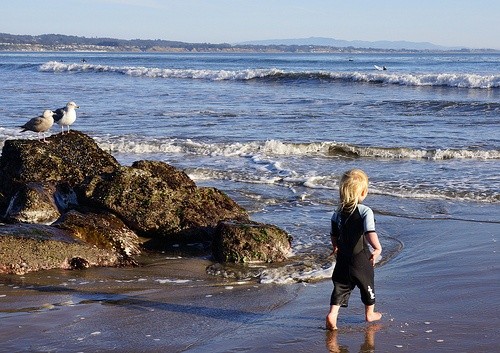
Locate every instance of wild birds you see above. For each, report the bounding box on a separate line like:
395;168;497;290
17;110;57;144
53;101;80;135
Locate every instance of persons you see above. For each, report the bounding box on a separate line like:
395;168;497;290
324;170;384;328
325;323;385;353
